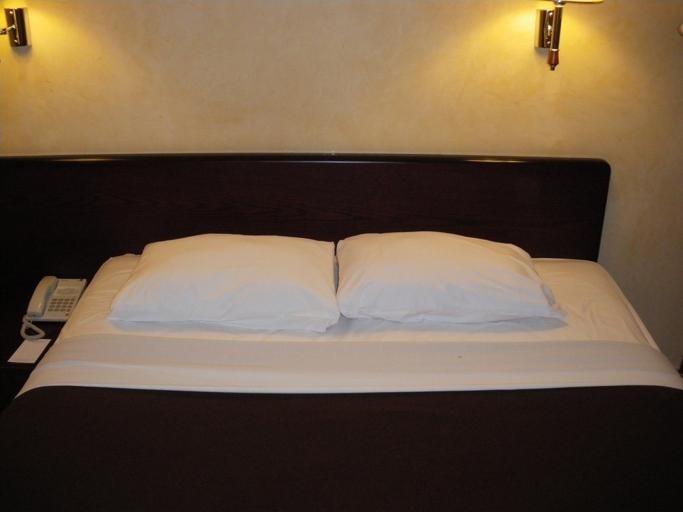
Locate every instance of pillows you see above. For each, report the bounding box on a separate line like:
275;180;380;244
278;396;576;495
332;231;569;335
110;230;342;336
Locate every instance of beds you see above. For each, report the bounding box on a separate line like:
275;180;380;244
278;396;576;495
2;250;683;512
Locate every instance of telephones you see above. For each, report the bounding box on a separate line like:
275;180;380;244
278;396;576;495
20;276;88;341
